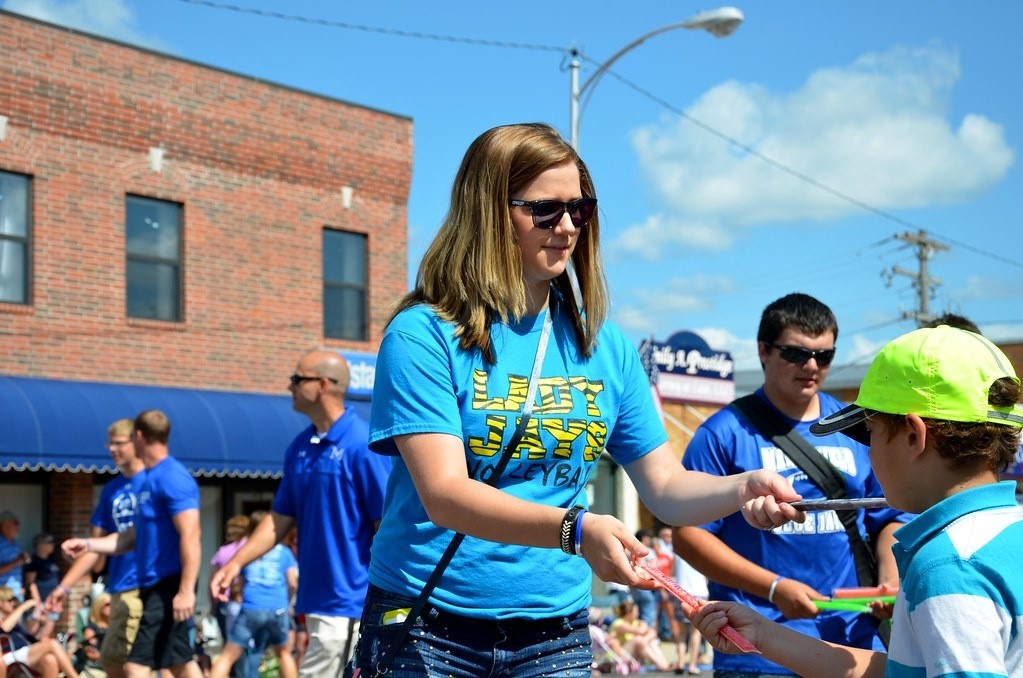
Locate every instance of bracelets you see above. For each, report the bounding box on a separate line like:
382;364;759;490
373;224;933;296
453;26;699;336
58;585;70;596
575;510;588;558
559;505;585;555
84;537;91;553
769;576;786;602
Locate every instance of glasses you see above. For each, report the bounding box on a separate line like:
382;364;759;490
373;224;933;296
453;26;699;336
108;440;131;446
290;374;338;386
508;197;597;230
770;342;835;367
8;599;13;602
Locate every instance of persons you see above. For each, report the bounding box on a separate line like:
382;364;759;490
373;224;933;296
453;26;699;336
0;509;309;678
46;419;147;678
672;294;921;678
682;323;1023;678
343;123;805;678
62;411;204;678
925;313;1023;500
211;350;396;678
587;528;713;678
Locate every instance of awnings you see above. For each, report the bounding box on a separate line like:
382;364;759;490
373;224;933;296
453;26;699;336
0;376;372;480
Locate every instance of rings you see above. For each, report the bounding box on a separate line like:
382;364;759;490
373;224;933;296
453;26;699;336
764;524;775;531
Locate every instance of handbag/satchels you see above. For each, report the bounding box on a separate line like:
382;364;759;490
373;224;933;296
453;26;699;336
343;632;388;678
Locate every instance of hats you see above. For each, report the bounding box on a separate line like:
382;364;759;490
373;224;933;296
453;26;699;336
809;325;1023;437
30;533;54;543
0;512;16;521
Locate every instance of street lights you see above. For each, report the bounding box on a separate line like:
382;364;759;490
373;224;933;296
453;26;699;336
564;6;746;152
